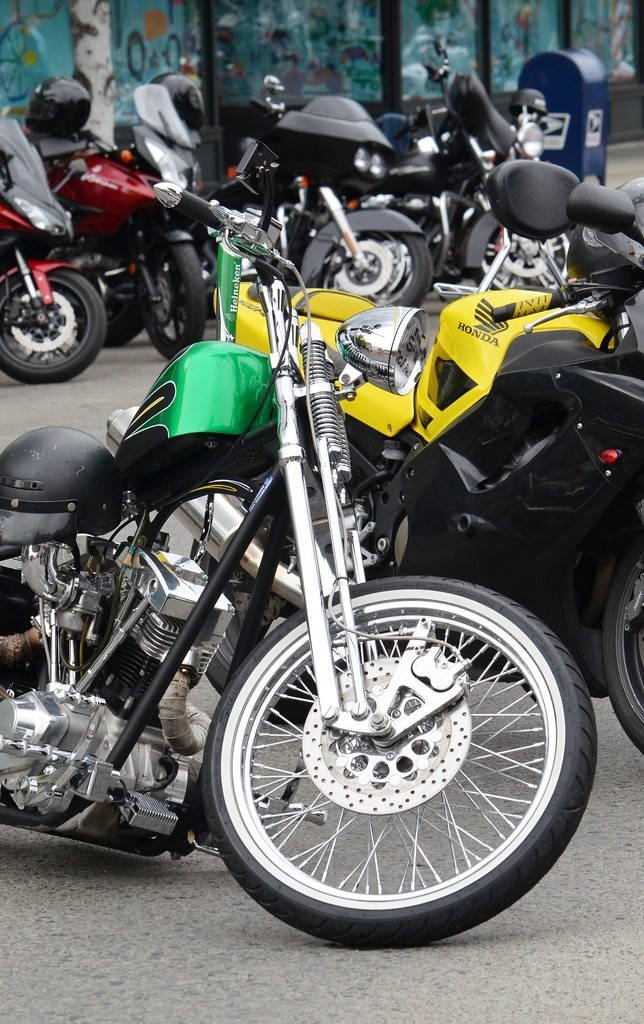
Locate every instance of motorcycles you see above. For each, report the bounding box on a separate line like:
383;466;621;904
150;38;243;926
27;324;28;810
0;116;107;384
31;37;549;359
213;160;644;756
1;181;597;952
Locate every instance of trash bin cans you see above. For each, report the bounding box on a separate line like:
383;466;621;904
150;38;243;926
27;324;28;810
518;45;611;184
